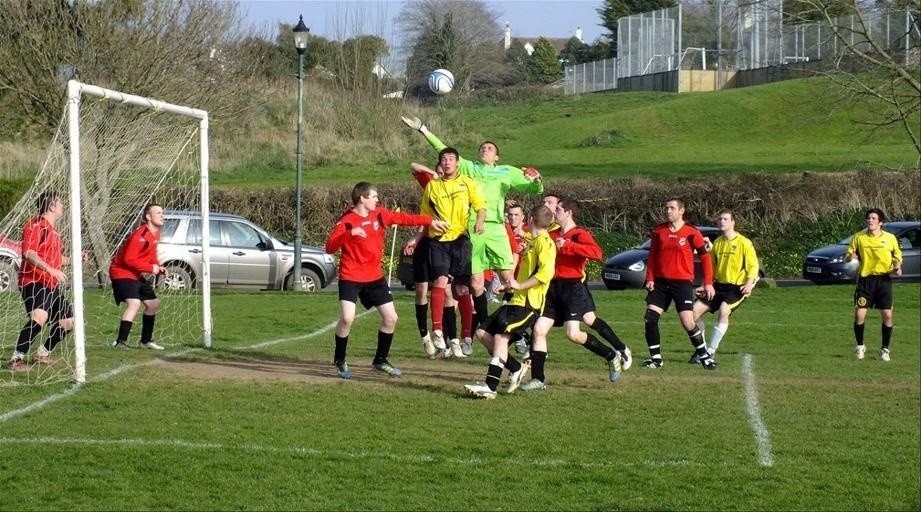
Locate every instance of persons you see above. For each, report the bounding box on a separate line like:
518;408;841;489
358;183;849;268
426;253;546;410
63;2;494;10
845;209;902;361
9;190;75;369
484;199;530;307
325;182;450;376
541;197;633;371
520;194;620;392
405;160;464;360
640;197;717;370
463;205;556;401
401;114;544;330
689;208;759;363
110;205;169;349
403;147;487;355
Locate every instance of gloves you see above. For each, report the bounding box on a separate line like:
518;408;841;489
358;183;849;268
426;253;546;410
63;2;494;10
522;166;541;183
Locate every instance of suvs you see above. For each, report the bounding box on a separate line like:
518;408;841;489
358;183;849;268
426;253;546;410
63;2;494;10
143;213;336;292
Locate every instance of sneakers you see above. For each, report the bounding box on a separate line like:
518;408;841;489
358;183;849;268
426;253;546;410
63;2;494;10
10;359;33;372
508;362;528;393
464;384;497;400
607;351;621;382
432;328;446;350
640;359;664;368
879;348;889;362
33;355;57;365
687;350;699;363
422;330;435;355
855;344;865;360
446;336;463;358
618;345;633;370
700;356;716;369
139;341;165;351
520;378;544;391
430;348;453;359
373;359;401;378
523;353;548;367
694;352;713;363
461;336;473;355
112;340;131;350
334;359;352;379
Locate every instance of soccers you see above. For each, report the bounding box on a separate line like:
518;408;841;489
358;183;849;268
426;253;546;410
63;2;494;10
428;69;454;94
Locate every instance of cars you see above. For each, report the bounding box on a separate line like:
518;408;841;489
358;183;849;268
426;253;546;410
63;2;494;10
803;222;921;285
602;226;765;290
0;235;31;291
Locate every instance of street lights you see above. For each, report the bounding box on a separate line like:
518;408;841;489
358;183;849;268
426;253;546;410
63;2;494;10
292;13;310;294
